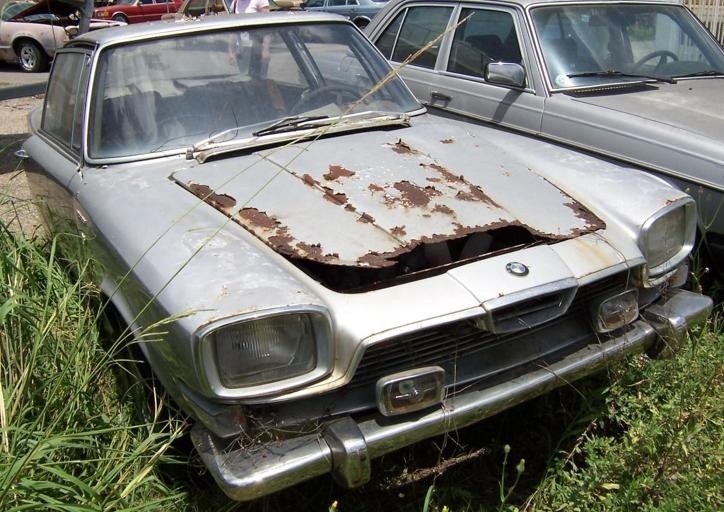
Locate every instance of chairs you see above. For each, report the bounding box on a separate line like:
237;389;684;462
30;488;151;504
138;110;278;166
99;78;285;152
466;35;577;85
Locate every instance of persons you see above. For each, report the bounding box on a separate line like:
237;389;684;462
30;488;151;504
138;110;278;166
229;0;272;81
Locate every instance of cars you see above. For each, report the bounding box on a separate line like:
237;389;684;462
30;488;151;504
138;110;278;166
298;0;721;237
12;9;713;502
1;1;388;73
